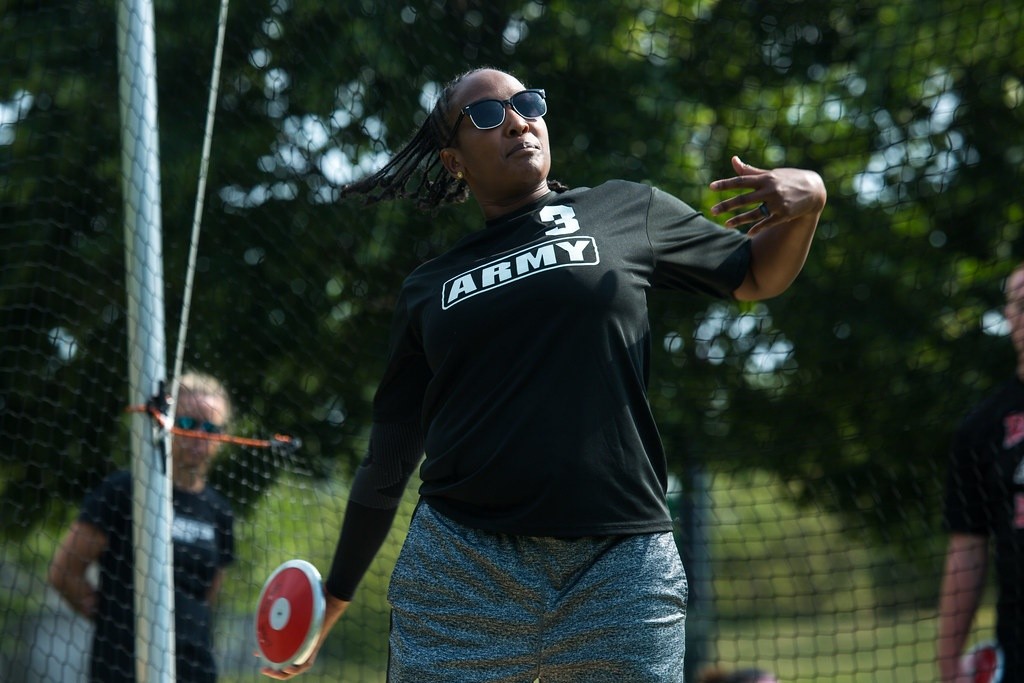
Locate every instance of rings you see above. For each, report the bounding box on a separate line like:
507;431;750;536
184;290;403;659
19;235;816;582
759;202;770;218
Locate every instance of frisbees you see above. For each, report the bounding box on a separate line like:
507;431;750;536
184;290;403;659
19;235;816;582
956;643;1005;683
252;557;328;670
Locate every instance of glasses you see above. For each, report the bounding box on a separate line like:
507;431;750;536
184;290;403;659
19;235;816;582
445;89;547;149
174;416;228;434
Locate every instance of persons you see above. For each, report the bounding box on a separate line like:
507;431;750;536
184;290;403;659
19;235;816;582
256;67;826;681
52;370;237;683
933;266;1023;683
